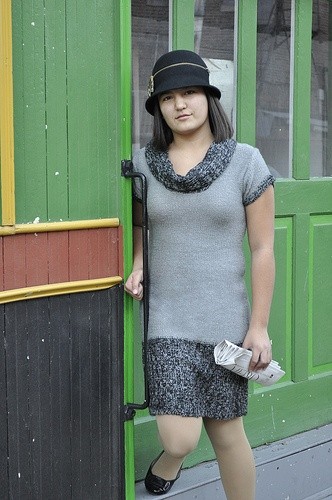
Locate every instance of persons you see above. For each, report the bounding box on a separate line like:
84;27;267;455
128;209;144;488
122;49;276;500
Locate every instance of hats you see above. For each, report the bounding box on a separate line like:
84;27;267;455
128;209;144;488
145;50;221;116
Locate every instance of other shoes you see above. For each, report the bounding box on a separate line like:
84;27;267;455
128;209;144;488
145;450;183;495
